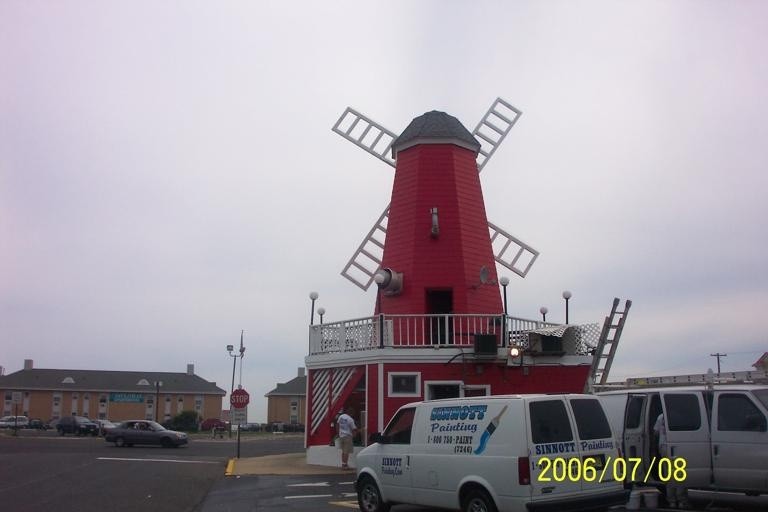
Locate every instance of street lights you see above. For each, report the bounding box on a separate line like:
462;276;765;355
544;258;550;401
154;381;162;423
540;307;547;321
500;277;509;315
374;274;384;314
309;291;325;325
562;291;571;324
227;345;245;438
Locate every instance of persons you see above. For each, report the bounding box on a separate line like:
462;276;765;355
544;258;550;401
654;410;690;509
334;406;358;470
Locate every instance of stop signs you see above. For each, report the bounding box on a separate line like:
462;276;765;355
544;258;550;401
230;390;249;408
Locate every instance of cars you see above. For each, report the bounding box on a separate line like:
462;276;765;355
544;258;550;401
239;422;261;432
0;416;187;448
199;418;229;431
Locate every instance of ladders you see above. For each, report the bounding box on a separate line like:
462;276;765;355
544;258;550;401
583;297;632;394
